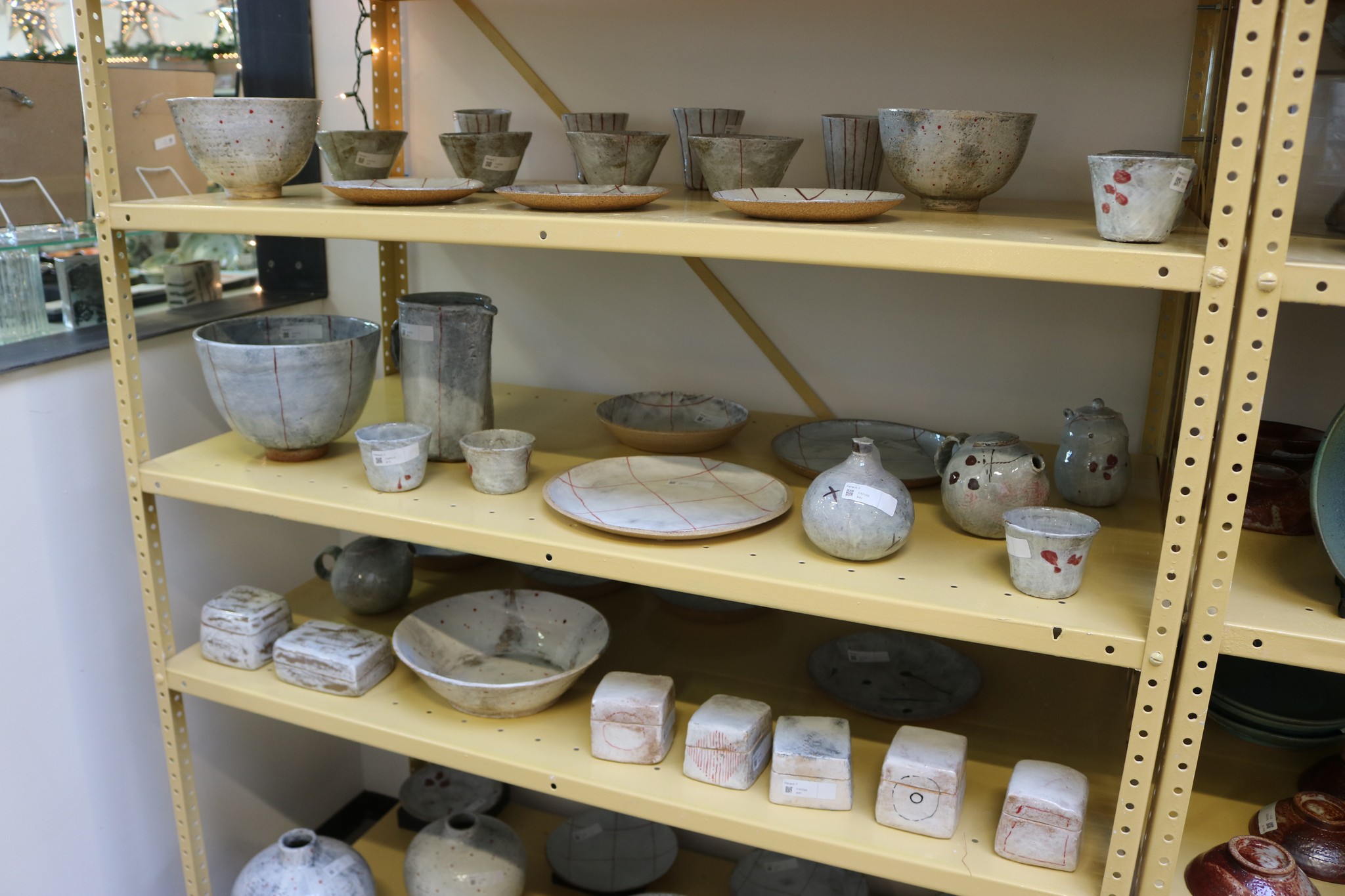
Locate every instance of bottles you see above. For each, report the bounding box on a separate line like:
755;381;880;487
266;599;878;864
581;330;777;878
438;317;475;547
230;829;376;896
800;436;915;559
1054;397;1131;506
403;810;529;896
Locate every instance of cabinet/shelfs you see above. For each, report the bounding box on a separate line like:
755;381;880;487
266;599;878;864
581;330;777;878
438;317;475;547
71;1;1279;896
1133;1;1345;896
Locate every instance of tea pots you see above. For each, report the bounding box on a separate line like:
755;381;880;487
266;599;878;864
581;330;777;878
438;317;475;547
934;432;1052;540
313;536;416;614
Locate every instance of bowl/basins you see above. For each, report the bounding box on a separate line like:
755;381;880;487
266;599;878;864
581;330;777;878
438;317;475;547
877;108;1038;208
191;315;380;461
166;95;322;199
391;588;610;718
1184;749;1345;896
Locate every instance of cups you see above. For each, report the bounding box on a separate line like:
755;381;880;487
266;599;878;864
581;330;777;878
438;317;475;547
320;107;883;201
1001;506;1100;600
353;423;537;495
1088;154;1195;243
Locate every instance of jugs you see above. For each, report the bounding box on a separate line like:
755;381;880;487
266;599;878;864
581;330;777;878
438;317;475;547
389;290;498;461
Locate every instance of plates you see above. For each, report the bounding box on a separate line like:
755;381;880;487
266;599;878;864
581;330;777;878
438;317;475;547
1207;655;1345;749
1310;405;1345;583
730;847;871;896
324;176;907;223
540;391;950;540
806;630;981;722
545;810;677;893
399;764;504;821
